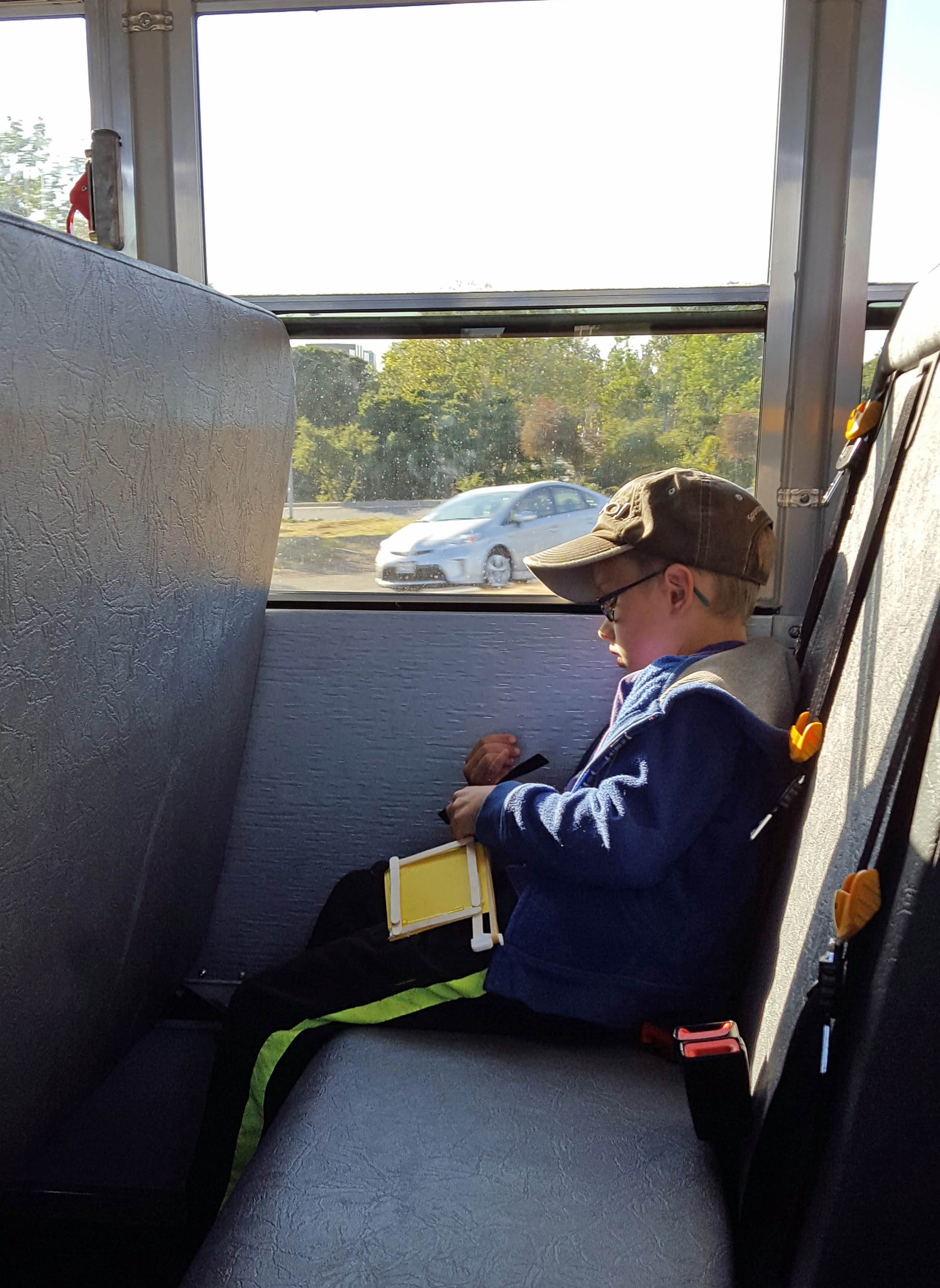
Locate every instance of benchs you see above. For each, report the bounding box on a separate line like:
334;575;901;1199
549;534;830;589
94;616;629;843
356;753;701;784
0;210;940;1288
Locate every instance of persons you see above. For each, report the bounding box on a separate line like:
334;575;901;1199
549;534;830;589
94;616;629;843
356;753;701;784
137;465;802;1254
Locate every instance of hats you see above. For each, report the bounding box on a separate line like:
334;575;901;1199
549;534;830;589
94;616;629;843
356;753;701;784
522;467;774;604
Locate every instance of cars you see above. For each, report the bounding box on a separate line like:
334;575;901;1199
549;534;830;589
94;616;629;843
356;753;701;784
375;480;613;591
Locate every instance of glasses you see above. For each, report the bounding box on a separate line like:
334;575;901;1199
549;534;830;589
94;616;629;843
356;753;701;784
596;566;709;622
